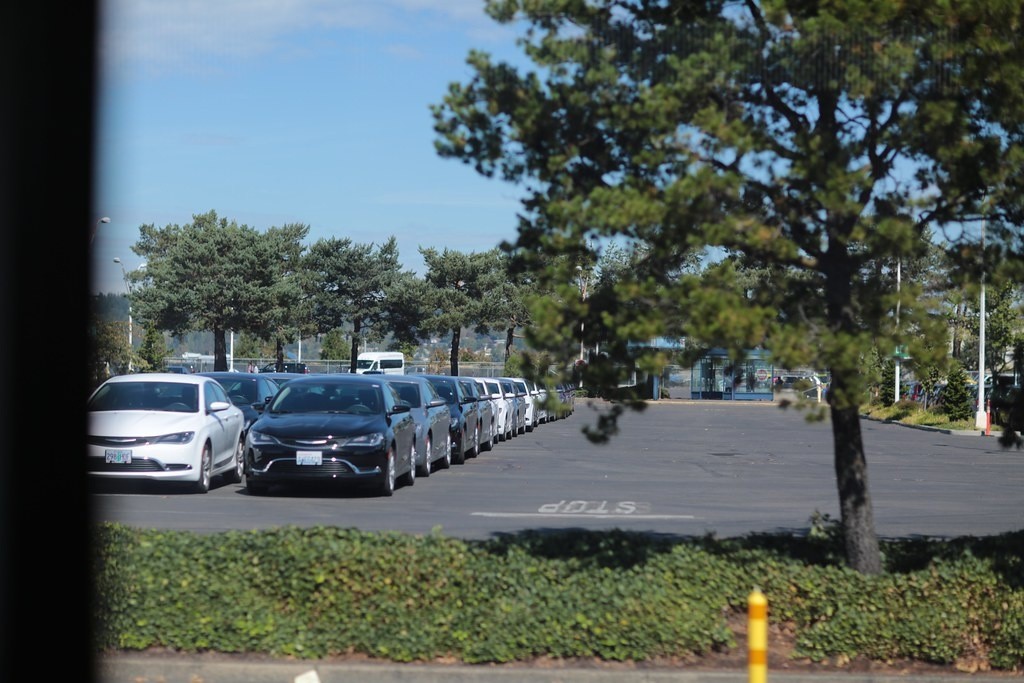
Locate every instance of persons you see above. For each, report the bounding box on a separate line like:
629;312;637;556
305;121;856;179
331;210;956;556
774;377;783;383
248;362;257;373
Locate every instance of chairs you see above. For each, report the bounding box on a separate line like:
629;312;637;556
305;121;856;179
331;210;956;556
358;388;377;410
113;390;143;409
299;392;328;410
399;386;419;408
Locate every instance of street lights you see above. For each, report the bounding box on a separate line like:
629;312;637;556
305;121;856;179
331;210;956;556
113;258;147;374
576;266;600;390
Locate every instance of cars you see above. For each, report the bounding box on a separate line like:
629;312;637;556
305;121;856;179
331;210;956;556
907;371;1021;404
366;374;574;478
88;373;244;495
243;374;417;497
670;375;821;389
167;367;309;442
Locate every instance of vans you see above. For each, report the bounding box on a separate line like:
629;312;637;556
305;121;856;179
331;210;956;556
259;363;309;374
347;352;405;374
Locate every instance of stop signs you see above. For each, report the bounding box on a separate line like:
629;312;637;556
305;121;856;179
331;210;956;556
757;369;767;380
